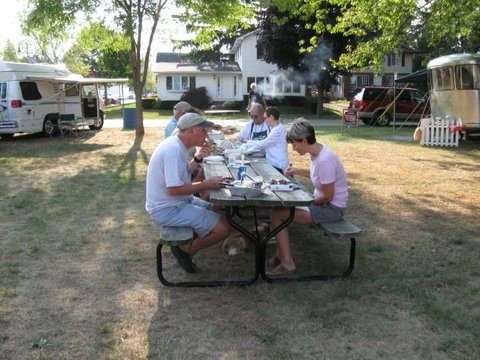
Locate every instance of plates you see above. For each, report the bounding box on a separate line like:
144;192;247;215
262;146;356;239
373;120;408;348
269;182;300;189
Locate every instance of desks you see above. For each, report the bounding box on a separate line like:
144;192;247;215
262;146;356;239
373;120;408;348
199;140;313;283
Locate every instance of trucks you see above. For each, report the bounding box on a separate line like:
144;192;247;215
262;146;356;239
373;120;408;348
0;61;105;140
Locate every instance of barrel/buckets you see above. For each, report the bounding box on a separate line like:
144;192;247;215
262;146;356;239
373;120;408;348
123;107;136;128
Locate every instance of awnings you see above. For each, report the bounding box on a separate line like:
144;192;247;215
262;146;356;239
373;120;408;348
393;69;427;83
30;76;128;83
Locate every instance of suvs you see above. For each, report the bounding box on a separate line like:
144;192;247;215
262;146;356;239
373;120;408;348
349;86;431;126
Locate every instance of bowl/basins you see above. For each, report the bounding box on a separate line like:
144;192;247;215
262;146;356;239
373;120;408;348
229;181;266;197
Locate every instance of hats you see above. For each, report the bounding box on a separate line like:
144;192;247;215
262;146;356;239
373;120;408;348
177;112;214;131
174;102;192;119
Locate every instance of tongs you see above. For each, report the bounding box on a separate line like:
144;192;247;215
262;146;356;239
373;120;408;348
240;172;255;187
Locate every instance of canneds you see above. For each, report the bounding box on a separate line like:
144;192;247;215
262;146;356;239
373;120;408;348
237;165;246;181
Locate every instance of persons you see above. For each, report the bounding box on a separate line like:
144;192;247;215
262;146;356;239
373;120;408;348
242;107;290;178
247;81;265;107
144;112;231;275
163;100;214;146
231;103;270;143
264;118;350;275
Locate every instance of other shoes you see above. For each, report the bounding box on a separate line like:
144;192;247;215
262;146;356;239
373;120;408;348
171;246;196;273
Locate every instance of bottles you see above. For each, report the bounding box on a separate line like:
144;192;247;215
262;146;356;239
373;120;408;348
211;143;217;156
237;154;247;180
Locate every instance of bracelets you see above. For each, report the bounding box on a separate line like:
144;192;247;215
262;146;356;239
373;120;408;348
194;156;203;164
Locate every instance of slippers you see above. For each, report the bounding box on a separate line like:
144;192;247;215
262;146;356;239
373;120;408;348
266;264;295;276
265;256;281;269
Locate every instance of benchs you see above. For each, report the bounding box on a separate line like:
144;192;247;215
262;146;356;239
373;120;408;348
160;173;362;246
